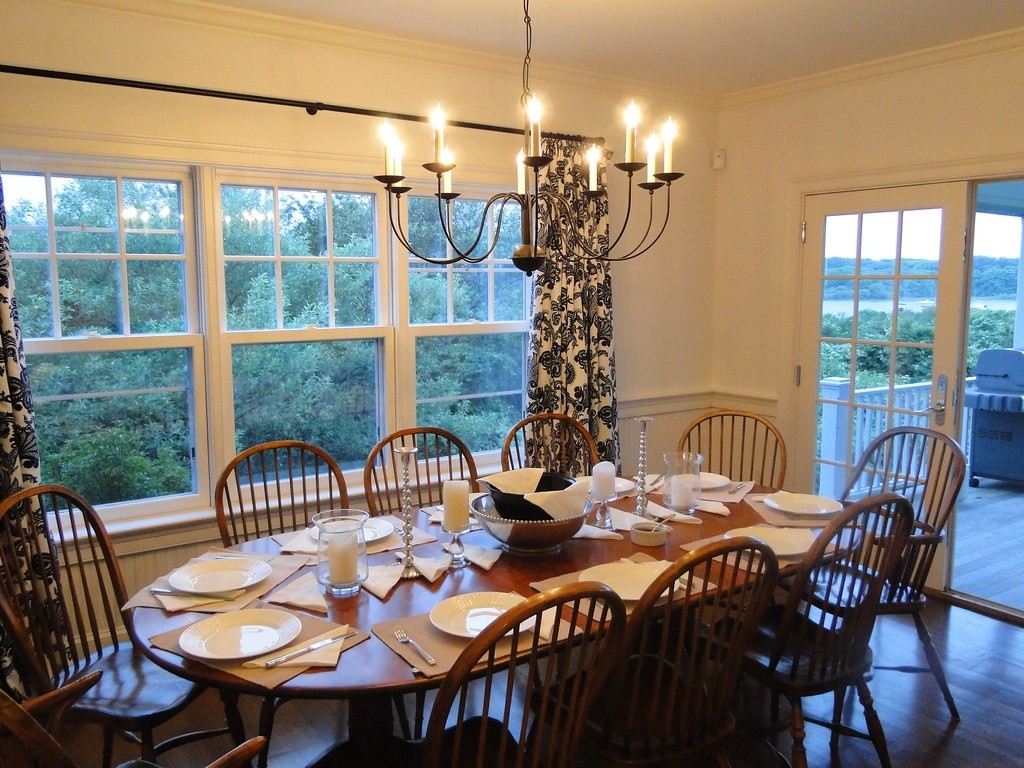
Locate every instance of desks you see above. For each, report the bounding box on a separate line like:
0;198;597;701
133;477;870;743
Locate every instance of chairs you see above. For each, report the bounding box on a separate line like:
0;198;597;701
524;536;778;768
213;440;412;768
712;492;914;768
0;670;266;768
676;408;787;491
501;413;599;477
0;485;252;768
393;581;627;768
365;427;546;741
778;425;967;747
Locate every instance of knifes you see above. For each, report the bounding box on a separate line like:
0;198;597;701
265;632;358;670
150;588;235;602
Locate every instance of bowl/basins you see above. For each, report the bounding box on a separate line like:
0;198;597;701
631;521;667;547
469;472;595;553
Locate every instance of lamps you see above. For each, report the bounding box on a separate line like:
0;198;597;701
372;0;687;278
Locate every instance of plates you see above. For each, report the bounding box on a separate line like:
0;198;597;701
578;562;680;601
310;518;394;544
469;493;488;515
669;472;732;490
178;608;302;660
724;527;813;556
575;476;636;494
169;557;272;594
430;591;537;638
764;493;843;515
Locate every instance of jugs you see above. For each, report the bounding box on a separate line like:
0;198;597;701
662;452;704;516
312;509;370;596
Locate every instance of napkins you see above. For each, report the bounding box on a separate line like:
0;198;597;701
123;473;859;768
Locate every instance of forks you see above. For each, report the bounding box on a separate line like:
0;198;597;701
784;512;833;521
728;484;744;494
215;554;275;563
392;624;437;666
